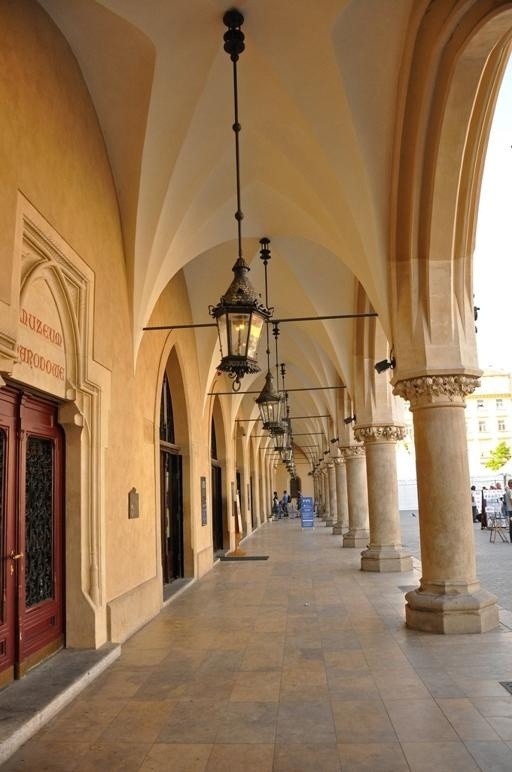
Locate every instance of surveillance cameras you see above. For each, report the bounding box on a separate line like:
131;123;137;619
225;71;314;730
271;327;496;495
308;449;330;475
331;438;339;443
375;360;394;374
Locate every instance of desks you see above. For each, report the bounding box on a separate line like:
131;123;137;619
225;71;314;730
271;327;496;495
490;512;508;544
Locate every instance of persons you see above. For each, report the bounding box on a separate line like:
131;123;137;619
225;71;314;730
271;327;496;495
296;490;302;517
273;490;289;520
471;479;512;543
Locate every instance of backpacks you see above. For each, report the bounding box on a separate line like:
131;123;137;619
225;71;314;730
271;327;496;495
288;495;291;503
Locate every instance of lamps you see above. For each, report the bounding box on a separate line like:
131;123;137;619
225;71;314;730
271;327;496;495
209;8;275;393
330;438;339;443
254;236;285;432
269;318;296;482
324;448;330;455
374;342;396;374
